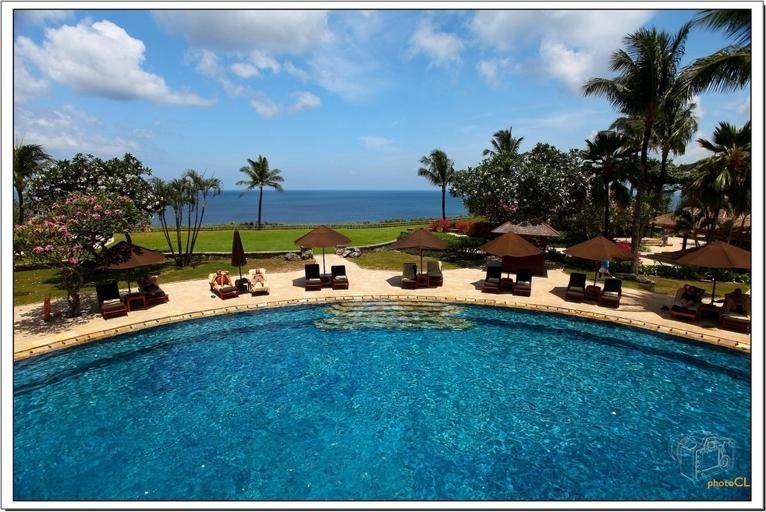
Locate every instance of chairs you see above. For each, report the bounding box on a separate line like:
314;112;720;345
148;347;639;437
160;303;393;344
672;287;751;335
401;260;444;290
208;264;350;300
96;274;168;320
482;264;623;309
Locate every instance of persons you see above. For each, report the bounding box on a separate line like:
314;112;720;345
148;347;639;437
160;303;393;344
210;270;233;287
725;288;745;313
598;260;616;280
681;286;698;308
251;268;265;287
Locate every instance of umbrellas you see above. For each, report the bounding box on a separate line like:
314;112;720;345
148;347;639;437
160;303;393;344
295;225;353;275
480;231;544;279
93;241;165;297
562;235;636;287
390;229;449;275
675;240;751;305
231;230;247;279
